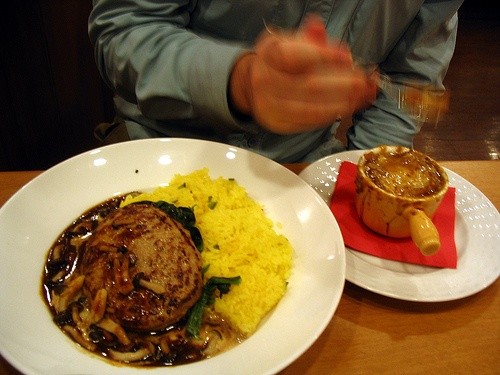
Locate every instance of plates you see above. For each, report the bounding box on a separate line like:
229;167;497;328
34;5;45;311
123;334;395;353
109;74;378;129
295;148;500;304
0;138;345;375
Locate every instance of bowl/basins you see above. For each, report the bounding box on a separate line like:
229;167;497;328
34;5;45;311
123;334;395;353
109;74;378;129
355;145;449;257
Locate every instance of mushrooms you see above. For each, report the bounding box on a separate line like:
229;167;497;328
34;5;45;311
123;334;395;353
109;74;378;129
41;207;205;365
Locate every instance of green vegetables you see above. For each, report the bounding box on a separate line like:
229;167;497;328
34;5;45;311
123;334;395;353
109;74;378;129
127;202;241;339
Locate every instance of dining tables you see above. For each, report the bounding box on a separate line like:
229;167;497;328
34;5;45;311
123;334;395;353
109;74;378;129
0;161;500;375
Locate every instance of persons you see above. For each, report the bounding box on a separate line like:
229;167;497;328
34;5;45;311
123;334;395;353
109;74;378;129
87;0;465;163
